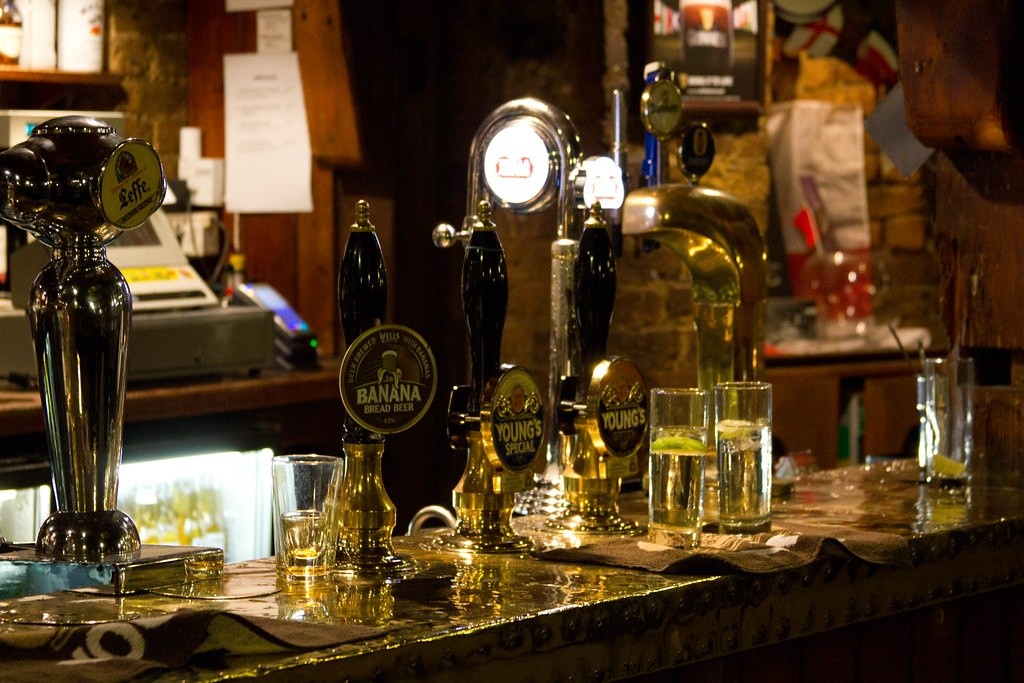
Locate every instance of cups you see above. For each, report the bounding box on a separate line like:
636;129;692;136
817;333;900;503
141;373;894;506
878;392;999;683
811;249;878;343
647;387;711;549
915;357;977;487
708;382;774;536
269;452;344;608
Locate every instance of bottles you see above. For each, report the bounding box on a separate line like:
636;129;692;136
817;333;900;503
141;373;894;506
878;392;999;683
0;0;23;64
